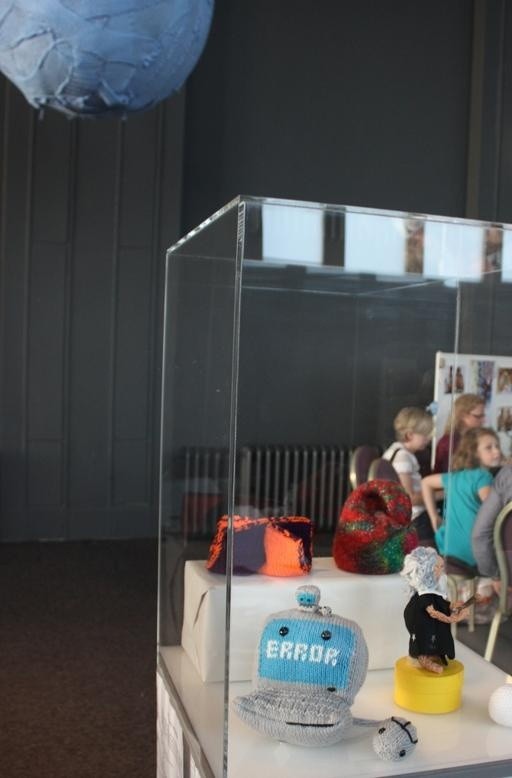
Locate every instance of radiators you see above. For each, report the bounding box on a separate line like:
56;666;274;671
177;446;389;546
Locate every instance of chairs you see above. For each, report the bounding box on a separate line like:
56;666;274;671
350;442;511;662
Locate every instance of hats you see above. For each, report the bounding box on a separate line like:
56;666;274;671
232;586;419;764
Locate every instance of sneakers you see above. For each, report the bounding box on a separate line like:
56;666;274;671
475;608;507;624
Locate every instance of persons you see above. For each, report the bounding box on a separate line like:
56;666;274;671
383;406;444;538
471;461;512;601
432;392;486;474
421;427;502;577
399;547;471;673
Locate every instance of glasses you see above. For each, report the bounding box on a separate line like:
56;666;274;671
470;412;485;418
